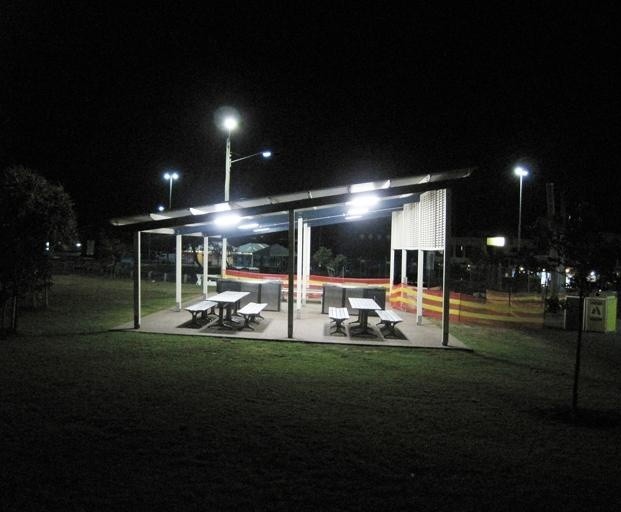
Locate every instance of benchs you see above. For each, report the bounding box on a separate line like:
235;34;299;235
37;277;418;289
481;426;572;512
184;301;268;331
328;307;404;339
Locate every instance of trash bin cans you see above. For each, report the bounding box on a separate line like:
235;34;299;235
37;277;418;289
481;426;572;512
585;291;618;336
564;291;590;331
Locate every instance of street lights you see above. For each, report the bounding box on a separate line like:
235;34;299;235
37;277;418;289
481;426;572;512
163;172;179;210
514;165;529;293
569;271;596;418
220;115;271;281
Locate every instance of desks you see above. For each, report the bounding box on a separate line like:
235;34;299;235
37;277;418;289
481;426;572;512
348;297;381;338
206;290;251;330
